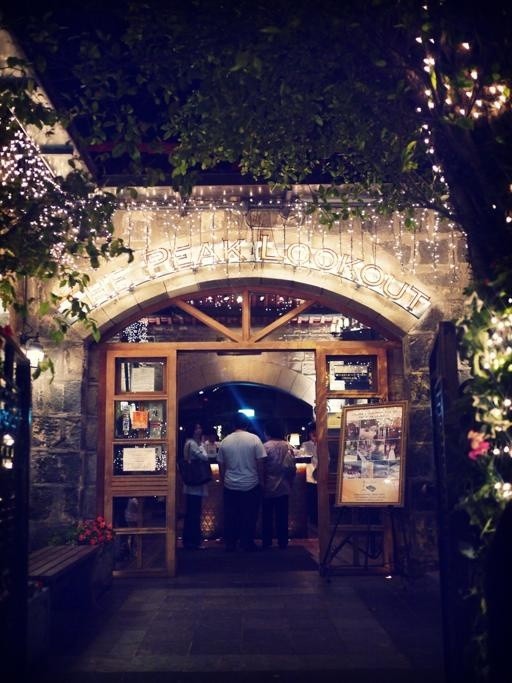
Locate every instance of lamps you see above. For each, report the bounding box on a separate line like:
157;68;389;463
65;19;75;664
286;434;300;451
21;330;47;370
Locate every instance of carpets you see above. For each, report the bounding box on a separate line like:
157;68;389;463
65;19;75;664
148;546;315;577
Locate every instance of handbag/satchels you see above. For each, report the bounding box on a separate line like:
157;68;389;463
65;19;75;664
178;443;212;486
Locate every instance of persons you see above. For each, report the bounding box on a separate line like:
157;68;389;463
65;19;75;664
301;422;331;534
216;412;268;547
262;422;297;549
182;422;208;551
204;430;219;454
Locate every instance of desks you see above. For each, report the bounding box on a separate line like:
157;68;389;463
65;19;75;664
155;456;314;540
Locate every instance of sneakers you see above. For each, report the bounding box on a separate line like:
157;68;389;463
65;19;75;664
182;542;288;554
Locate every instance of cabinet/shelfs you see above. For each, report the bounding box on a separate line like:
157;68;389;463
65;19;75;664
429;318;469;676
0;327;30;670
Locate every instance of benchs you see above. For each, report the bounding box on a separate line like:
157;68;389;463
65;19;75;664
27;540;100;620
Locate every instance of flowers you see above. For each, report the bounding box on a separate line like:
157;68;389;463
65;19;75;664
71;517;116;543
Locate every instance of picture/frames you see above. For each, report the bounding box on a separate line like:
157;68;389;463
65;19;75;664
338;398;412;510
